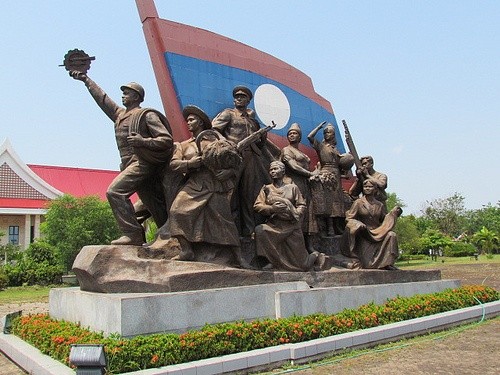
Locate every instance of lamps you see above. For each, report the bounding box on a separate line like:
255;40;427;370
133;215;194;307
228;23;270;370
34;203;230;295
70;344;107;375
2;310;22;334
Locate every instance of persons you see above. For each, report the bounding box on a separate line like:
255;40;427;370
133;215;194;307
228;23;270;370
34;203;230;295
254;160;330;271
210;85;268;238
348;155;388;213
345;178;403;271
307;120;355;236
278;122;322;261
58;48;174;245
162;104;255;270
